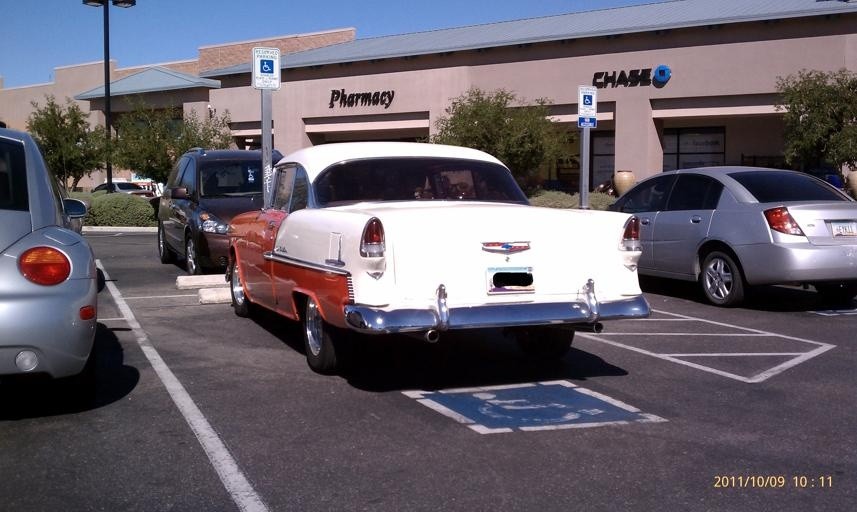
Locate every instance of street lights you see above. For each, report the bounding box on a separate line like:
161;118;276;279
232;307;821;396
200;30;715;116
79;0;138;194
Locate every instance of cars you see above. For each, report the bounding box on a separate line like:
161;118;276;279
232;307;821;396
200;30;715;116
604;166;855;309
225;140;650;379
0;128;106;386
92;182;155;199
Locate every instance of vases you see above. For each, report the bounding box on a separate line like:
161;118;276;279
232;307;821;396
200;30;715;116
613;171;636;197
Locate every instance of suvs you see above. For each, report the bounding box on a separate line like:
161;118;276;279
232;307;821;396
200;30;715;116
157;144;288;278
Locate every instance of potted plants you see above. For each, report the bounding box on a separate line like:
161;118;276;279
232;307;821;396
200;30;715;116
773;67;857;197
